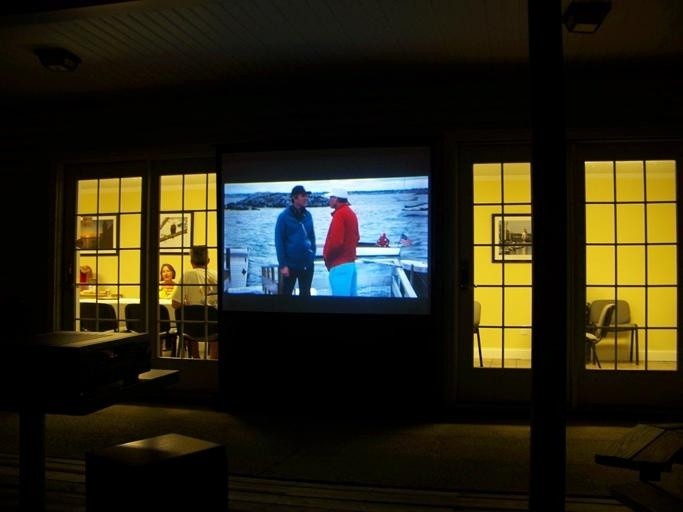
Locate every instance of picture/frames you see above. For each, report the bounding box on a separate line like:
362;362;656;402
157;210;194;255
79;214;120;256
491;214;533;263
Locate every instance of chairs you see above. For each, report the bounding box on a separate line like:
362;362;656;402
585;299;640;370
78;302;219;359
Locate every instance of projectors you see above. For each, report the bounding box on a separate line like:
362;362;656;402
1;331;151;396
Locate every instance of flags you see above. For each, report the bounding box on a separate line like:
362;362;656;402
400;234;411;247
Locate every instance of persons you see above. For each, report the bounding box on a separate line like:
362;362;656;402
274;185;316;295
376;233;390;248
172;245;217;340
322;188;360;296
159;264;176;298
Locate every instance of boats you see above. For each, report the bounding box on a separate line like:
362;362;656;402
314;239;401;258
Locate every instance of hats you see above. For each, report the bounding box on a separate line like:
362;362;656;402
292;185;311;196
328;186;352;205
